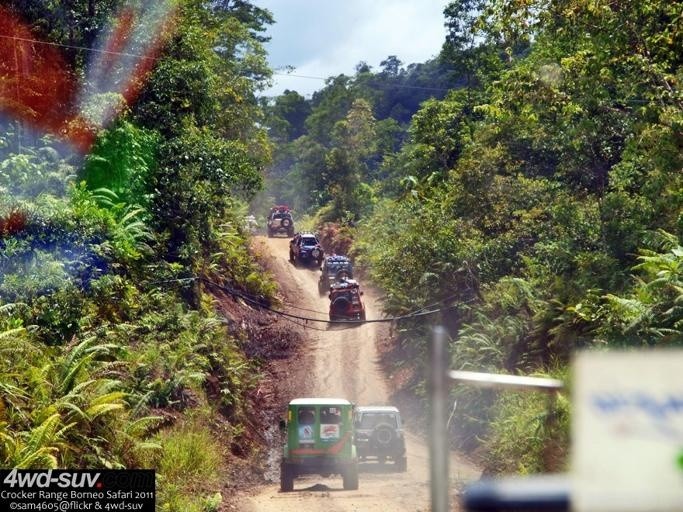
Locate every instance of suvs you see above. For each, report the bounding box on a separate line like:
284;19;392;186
267;206;295;238
290;233;366;324
281;397;407;490
